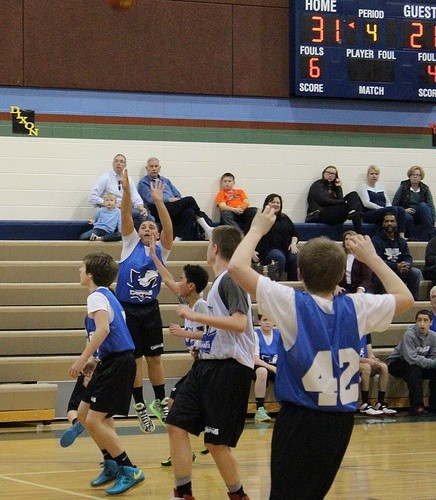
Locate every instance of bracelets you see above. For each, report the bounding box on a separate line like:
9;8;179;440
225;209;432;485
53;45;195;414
291;242;296;245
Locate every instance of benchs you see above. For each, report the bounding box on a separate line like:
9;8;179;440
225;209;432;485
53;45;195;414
0;218;436;425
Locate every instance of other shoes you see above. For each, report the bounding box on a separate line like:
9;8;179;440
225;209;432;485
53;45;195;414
204;228;214;240
173;487;194;500
90;233;97;240
227;491;249;500
348;212;363;220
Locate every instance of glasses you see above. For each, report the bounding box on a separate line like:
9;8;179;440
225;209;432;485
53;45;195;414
118;180;121;191
325;170;336;177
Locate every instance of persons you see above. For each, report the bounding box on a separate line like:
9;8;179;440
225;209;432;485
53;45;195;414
252;194;301;281
216;173;258;236
68;252;145;496
372;212;422;301
305;166;373;239
79;192;122;242
88;154;155;232
138;157;215;241
359;333;397;416
254;314;280;423
149;235;209;466
386;310;436;416
117;169;174;433
392;166;436;242
165;225;256;500
356;165;414;242
60;289;116;447
332;231;372;296
422;236;436;285
429;286;436;332
227;205;415;500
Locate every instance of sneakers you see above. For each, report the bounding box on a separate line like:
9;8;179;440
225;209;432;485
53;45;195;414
148;400;170;427
375;402;397;416
106;463;145;495
254;407;271;422
90;459;120;486
133;403;155;433
410;406;431;416
162;449;196;467
61;422;86;448
359;402;385;416
